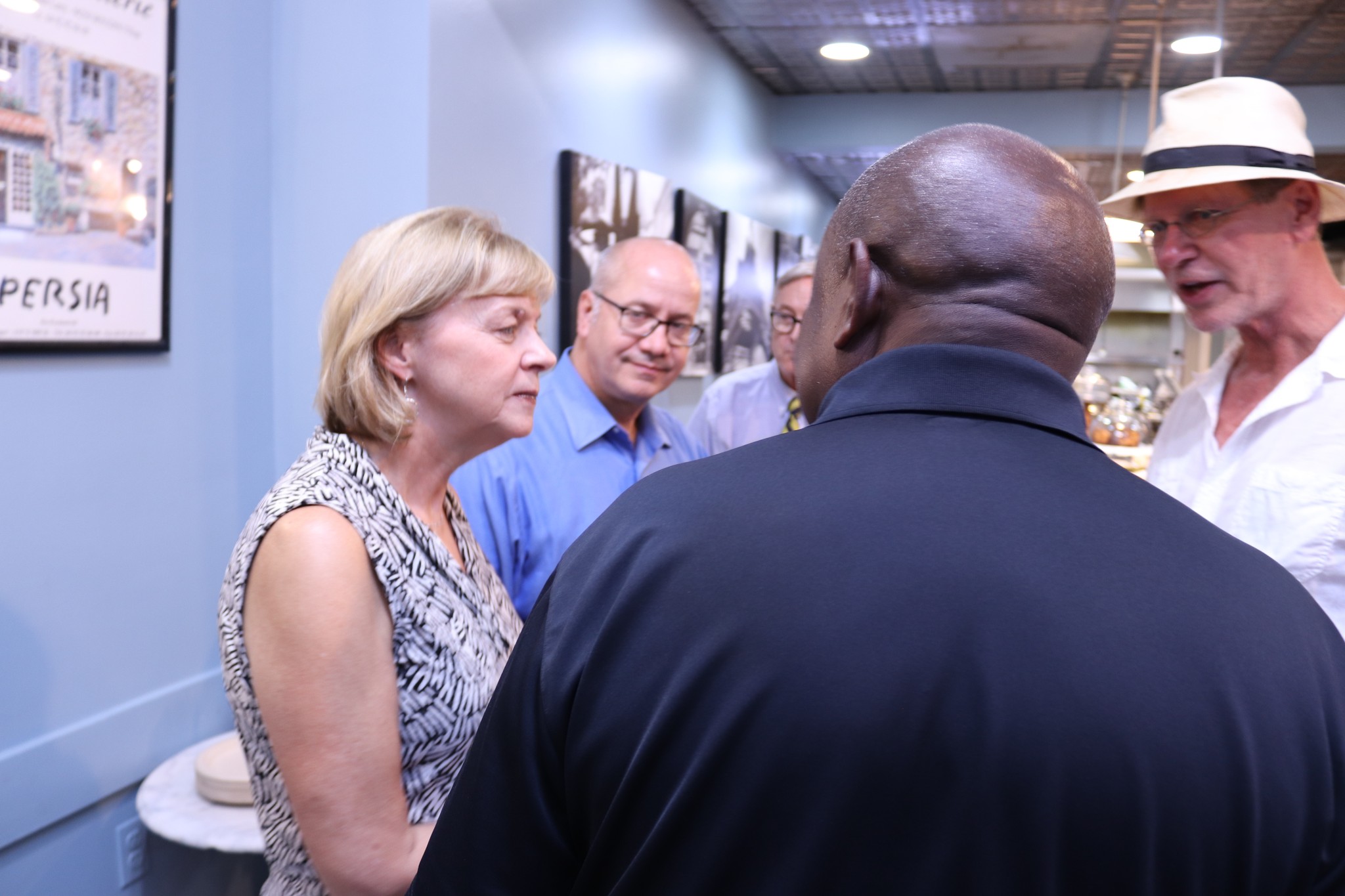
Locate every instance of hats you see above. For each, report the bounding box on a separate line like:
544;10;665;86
1095;77;1345;224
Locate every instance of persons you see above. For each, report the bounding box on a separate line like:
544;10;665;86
216;120;1345;896
1094;76;1345;643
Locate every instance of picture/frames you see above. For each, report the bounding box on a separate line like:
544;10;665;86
0;0;177;355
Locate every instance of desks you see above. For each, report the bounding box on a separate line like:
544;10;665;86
134;729;269;896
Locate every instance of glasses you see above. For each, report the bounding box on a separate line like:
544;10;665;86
769;310;802;335
595;290;705;348
1137;189;1282;246
